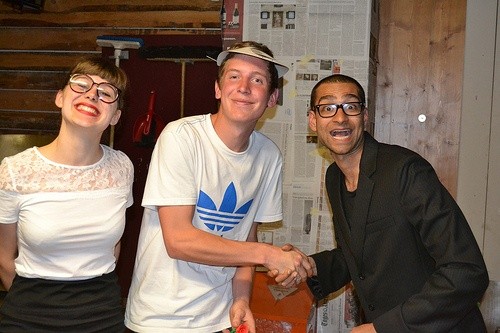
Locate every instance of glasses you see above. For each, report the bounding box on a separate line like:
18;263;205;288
69;73;121;109
312;102;365;118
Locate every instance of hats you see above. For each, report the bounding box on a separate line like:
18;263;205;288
216;46;289;79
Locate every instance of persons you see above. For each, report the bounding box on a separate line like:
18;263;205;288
0;58;134;333
125;41;313;333
266;75;489;333
